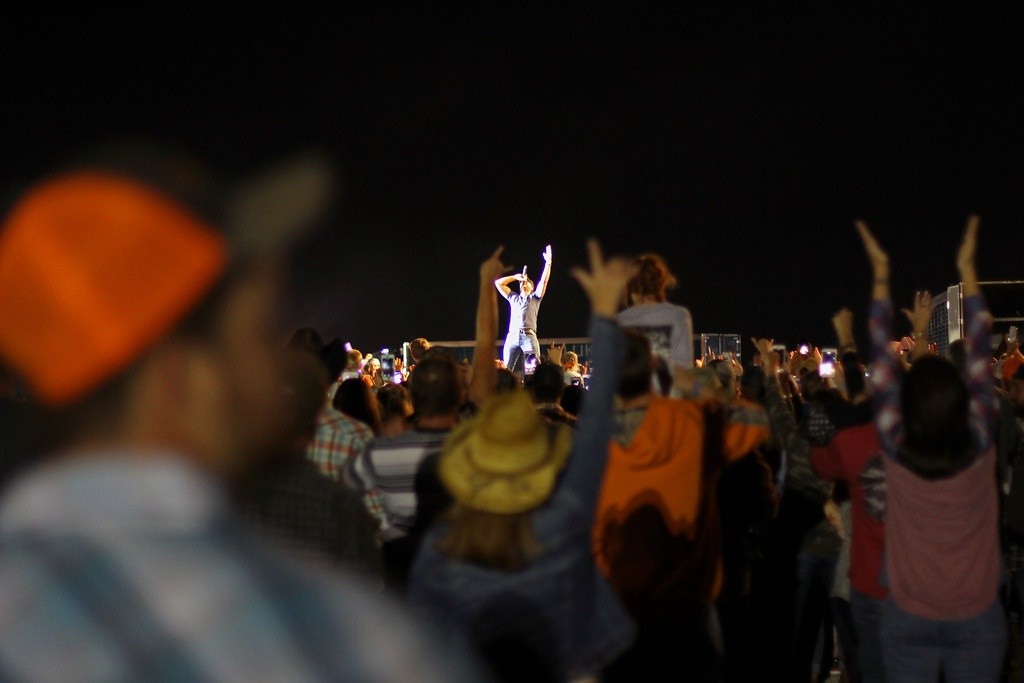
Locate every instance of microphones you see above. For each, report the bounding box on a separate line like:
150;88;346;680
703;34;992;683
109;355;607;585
522;265;527;278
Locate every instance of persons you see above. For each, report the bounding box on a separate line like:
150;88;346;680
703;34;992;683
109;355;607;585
0;167;475;682
495;244;552;382
259;219;1024;682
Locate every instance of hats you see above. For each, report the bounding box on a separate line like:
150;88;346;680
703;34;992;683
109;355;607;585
0;170;227;409
438;390;571;514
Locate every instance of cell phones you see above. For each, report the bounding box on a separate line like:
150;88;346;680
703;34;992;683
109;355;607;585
381;354;395;381
570;377;581;387
